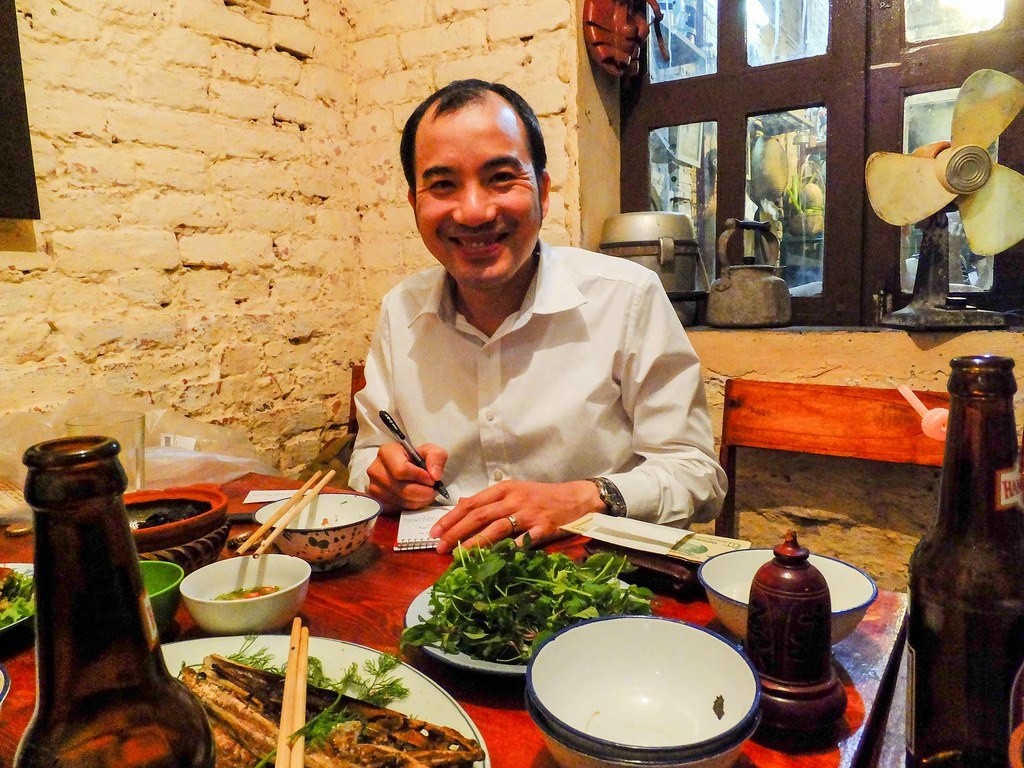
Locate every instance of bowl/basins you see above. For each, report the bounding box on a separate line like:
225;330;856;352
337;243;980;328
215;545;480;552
136;561;184;624
525;615;762;768
137;515;230;571
120;486;227;537
180;555;311;632
699;548;876;648
253;493;381;569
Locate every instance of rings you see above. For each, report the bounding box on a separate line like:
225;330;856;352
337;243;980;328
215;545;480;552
506;515;518;533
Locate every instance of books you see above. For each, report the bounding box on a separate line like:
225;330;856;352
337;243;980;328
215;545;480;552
394;505;459;551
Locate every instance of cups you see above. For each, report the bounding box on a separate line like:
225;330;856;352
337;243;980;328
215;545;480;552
65;410;145;501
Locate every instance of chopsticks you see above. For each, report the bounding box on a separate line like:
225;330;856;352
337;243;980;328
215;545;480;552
273;617;309;768
232;470;341;558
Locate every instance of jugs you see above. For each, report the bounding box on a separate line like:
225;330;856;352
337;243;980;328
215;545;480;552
707;220;792;327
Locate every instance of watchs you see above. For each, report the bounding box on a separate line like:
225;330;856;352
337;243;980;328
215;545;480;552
587;477;626;519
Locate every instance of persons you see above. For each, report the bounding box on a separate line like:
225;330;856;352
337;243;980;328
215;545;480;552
346;78;729;559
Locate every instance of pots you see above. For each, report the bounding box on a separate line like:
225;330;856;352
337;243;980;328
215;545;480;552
596;211;701;321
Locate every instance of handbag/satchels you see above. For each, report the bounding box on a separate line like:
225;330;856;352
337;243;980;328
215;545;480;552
297;433;356;490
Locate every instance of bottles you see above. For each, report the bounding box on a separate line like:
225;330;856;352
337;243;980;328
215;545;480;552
908;356;1024;768
14;436;215;768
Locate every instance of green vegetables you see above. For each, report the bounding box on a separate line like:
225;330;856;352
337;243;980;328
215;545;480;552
400;530;659;664
174;628;409;768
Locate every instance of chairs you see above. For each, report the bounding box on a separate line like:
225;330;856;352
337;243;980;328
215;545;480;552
714;378;951;537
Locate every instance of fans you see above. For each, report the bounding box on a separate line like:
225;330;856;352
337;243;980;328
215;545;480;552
865;69;1024;327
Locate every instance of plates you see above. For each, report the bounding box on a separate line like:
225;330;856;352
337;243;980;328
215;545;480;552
0;563;34;632
159;635;489;768
405;575;653;677
0;665;11;706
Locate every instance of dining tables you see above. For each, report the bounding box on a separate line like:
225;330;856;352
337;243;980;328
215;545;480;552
0;471;911;768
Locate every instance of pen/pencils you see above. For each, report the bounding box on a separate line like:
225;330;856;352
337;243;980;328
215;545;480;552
378;408;455;503
